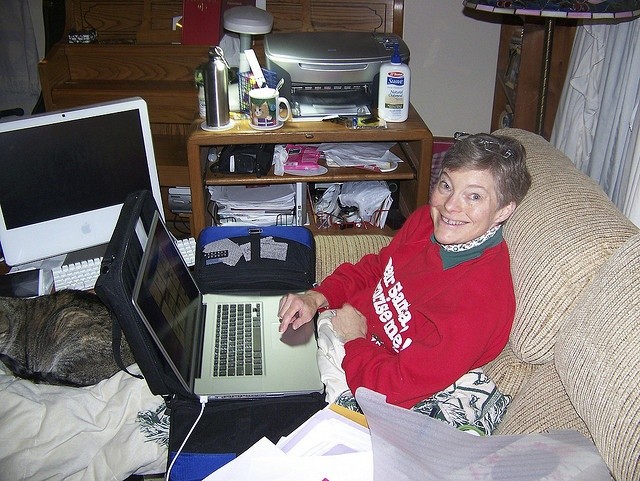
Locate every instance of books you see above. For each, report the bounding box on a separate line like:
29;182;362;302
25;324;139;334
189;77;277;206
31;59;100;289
180;0;221;47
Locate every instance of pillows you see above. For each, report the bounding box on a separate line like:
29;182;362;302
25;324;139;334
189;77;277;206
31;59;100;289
334;369;510;442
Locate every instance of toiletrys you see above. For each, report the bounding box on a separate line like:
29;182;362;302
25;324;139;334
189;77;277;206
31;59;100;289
377;42;410;123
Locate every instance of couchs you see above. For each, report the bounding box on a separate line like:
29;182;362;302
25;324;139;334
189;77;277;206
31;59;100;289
316;129;638;479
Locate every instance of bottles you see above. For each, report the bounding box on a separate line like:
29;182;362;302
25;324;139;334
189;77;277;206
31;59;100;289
202;45;229;127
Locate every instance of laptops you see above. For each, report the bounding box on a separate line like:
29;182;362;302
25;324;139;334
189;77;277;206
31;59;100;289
131;210;324;399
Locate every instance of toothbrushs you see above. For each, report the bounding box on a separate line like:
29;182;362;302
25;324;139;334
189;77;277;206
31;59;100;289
272;78;284;97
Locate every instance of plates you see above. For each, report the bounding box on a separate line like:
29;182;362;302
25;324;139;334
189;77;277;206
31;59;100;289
200;117;236;132
250;121;283;130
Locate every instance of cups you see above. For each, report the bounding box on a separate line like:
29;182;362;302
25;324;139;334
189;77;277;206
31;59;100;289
248;87;291;126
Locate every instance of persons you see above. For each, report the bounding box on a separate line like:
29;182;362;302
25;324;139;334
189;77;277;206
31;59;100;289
278;134;531;411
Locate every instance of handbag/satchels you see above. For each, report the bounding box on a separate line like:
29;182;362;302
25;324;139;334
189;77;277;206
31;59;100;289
195;224;315;291
95;190;327;481
210;144;274;176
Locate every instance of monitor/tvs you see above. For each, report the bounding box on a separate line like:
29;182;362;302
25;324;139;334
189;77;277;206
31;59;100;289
1;96;166;267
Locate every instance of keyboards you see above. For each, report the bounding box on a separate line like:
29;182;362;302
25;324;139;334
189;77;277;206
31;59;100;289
52;238;196;291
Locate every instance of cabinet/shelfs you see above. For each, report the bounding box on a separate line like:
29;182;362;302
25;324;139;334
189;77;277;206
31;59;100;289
38;29;211;220
186;98;434;238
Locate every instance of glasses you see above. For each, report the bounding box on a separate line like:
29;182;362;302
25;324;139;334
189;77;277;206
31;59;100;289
453;131;518;164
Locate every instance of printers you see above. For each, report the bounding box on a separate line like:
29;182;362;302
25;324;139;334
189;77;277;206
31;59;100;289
265;31;410;118
